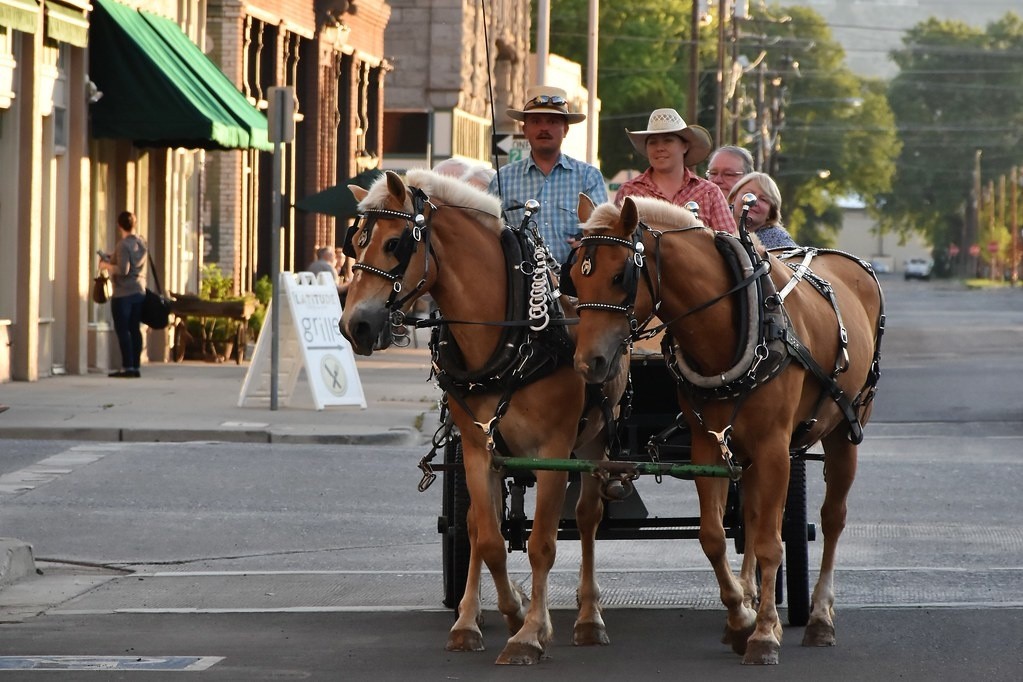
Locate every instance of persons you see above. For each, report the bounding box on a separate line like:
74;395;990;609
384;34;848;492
487;84;608;290
99;211;150;378
308;245;358;307
613;108;738;236
725;171;800;249
705;145;756;202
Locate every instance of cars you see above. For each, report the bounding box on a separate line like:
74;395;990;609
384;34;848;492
903;259;931;279
869;255;894;274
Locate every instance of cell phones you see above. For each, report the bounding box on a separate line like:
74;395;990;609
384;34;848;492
97;250;110;263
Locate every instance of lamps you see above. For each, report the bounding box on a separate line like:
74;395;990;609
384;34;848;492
85;74;103;104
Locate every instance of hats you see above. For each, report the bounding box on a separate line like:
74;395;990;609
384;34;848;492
506;86;586;124
625;107;713;167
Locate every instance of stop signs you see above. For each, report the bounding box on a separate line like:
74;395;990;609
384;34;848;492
950;246;960;255
970;245;980;256
989;241;999;252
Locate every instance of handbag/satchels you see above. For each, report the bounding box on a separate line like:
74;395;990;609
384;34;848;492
93;271;110;303
139;289;172;329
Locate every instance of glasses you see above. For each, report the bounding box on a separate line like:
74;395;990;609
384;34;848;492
524;95;569;112
705;170;745;180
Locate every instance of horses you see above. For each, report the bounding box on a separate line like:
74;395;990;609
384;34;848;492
570;188;884;666
337;166;632;665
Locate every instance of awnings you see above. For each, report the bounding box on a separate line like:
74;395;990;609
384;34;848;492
90;0;289;151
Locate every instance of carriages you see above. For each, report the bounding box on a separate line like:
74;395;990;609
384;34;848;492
335;168;887;671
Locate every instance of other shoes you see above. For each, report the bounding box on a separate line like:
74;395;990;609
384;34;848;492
135;370;141;377
108;369;135;377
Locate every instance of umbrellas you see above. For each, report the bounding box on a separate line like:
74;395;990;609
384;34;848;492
295;167;384;216
